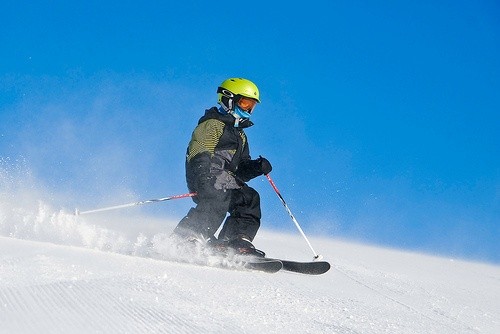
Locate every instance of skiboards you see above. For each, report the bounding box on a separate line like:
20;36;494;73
130;238;332;276
